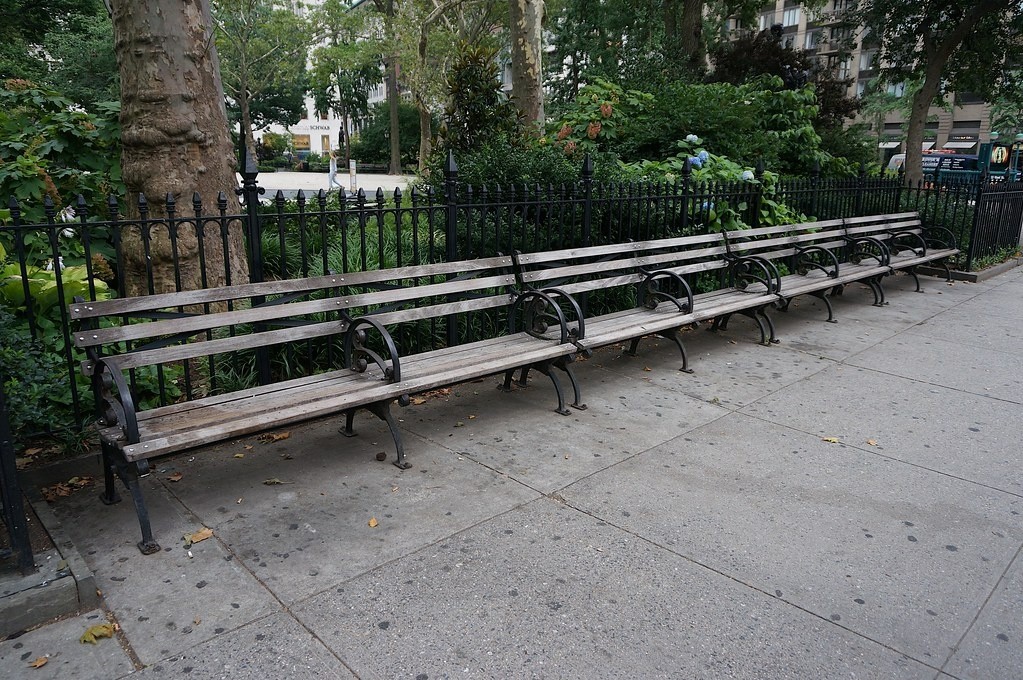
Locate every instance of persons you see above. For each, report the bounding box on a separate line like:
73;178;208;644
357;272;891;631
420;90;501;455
339;126;345;150
996;147;1003;163
282;146;293;163
328;150;345;192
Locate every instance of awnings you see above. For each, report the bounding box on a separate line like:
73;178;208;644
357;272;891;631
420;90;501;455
878;142;901;148
922;142;935;150
942;141;977;149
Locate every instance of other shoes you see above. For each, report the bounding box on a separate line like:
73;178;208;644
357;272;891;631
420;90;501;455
340;187;345;190
328;188;333;192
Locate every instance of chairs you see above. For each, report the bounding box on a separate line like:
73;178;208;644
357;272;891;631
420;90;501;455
68;198;963;560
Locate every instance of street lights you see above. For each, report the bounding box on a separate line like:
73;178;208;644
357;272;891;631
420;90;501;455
1012;133;1023;182
985;132;999;184
